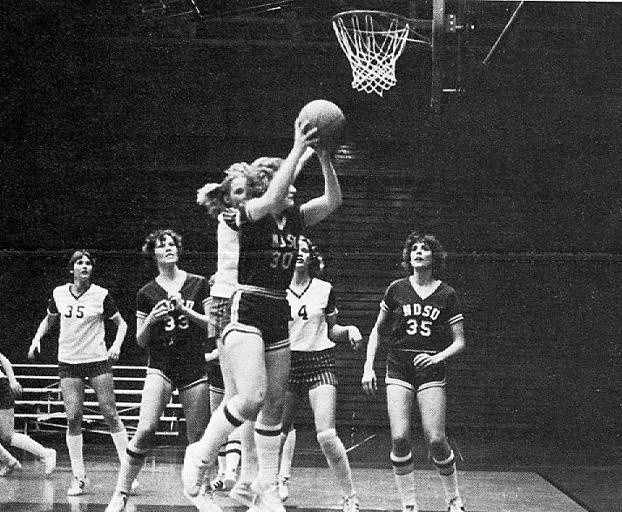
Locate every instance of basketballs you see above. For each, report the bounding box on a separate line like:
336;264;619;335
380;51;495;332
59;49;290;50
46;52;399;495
296;99;347;151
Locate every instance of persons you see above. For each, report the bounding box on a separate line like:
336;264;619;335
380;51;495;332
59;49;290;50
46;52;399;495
103;230;220;512
203;273;241;490
194;160;258;507
0;354;57;477
361;230;466;512
28;250;139;496
276;234;362;512
181;121;343;512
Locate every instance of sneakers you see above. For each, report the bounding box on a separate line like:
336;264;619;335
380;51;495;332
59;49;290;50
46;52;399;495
223;476;238;490
182;444;210;498
129;477;140;492
276;476;291;503
41;448;59;475
444;499;467;512
251;479;286;512
0;462;23;479
229;478;256;508
211;476;223;491
401;502;419;512
336;494;361;510
67;475;92;496
104;491;128;512
